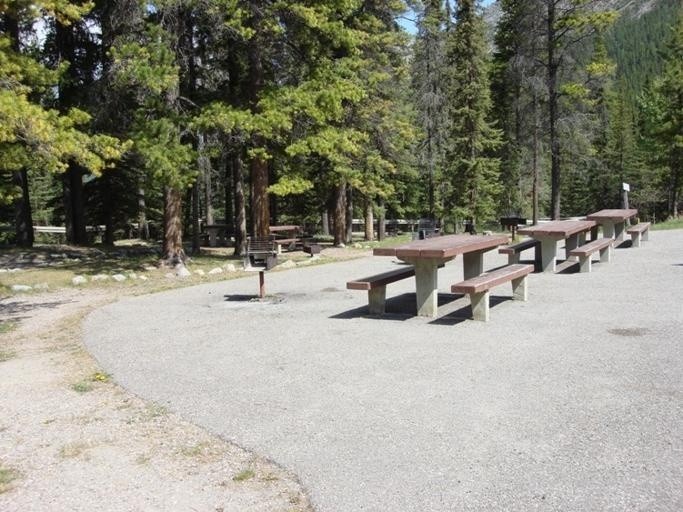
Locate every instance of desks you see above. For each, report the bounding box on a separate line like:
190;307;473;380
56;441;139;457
268;224;304;252
515;219;596;273
371;232;511;316
586;209;637;243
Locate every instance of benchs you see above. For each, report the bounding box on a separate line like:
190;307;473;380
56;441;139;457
625;222;650;248
271;238;302;254
498;238;541;274
345;261;446;317
579;227;597;245
450;262;534;320
570;238;615;272
